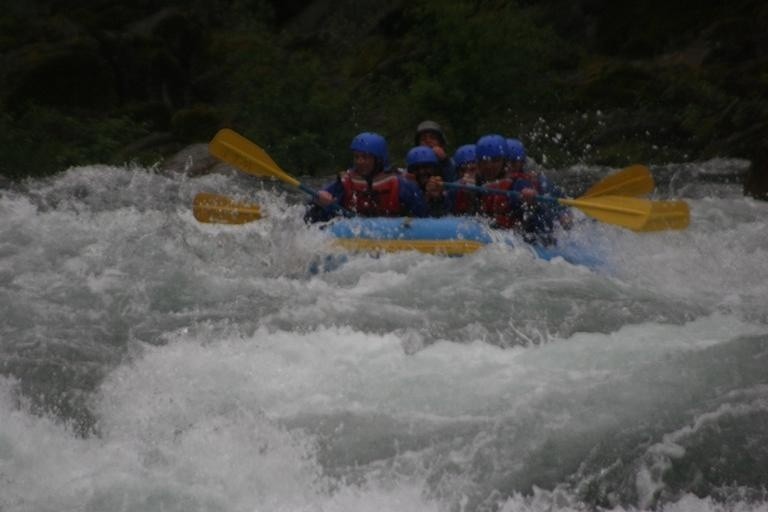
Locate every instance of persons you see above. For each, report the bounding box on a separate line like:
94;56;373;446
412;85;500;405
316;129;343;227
303;132;430;225
402;118;574;247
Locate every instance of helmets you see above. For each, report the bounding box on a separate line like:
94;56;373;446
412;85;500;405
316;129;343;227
349;133;388;159
406;147;439;172
415;121;442;145
455;134;525;169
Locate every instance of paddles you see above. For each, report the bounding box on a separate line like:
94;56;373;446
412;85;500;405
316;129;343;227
194;194;265;225
523;164;655;227
435;181;651;230
209;128;355;217
638;201;688;230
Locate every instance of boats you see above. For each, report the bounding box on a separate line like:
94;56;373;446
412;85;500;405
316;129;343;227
319;211;611;270
